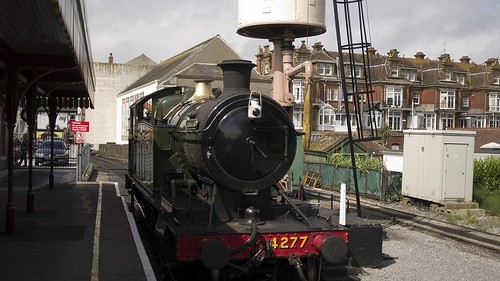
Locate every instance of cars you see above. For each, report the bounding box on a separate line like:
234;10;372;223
35;140;70;166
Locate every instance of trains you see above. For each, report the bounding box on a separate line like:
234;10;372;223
124;58;385;280
